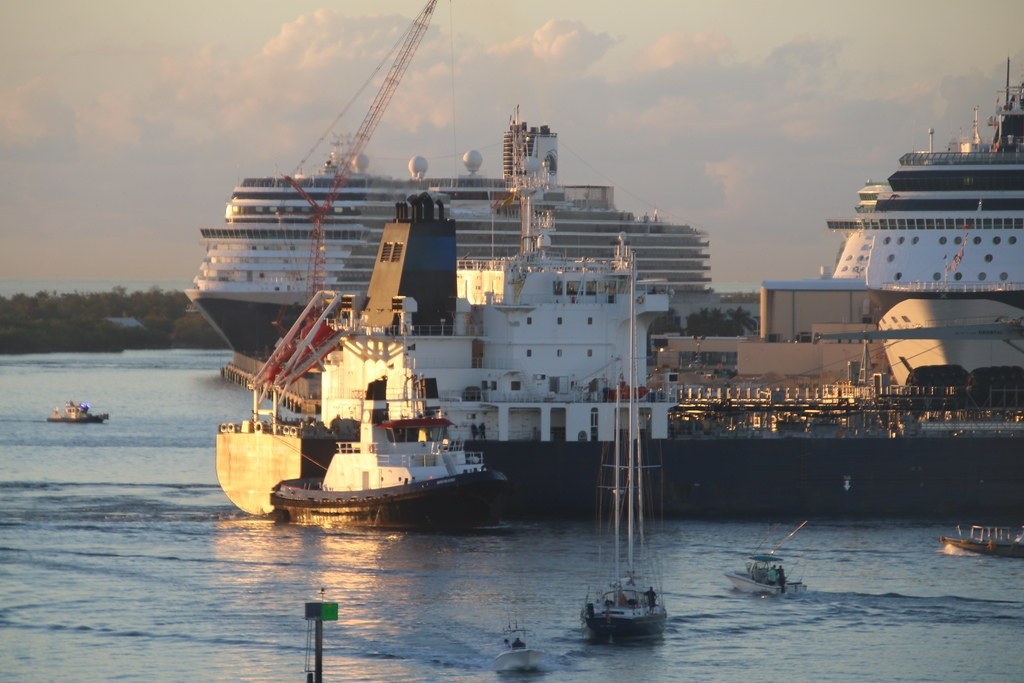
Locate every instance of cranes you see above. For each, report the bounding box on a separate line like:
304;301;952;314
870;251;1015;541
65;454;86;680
275;0;442;366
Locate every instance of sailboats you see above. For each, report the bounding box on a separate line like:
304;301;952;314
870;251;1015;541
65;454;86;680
576;246;670;640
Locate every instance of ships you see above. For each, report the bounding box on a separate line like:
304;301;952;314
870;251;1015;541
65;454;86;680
209;57;1023;527
186;163;722;357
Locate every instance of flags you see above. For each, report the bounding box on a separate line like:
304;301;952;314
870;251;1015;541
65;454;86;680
491;189;515;208
948;235;970;272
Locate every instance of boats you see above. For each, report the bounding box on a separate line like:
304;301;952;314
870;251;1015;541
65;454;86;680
44;398;110;425
264;367;508;535
938;524;1023;560
489;619;544;676
725;518;813;601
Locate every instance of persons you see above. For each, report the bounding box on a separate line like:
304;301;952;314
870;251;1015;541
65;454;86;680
471;421;488;441
510;638;527;650
776;565;785;590
644;587;657;616
